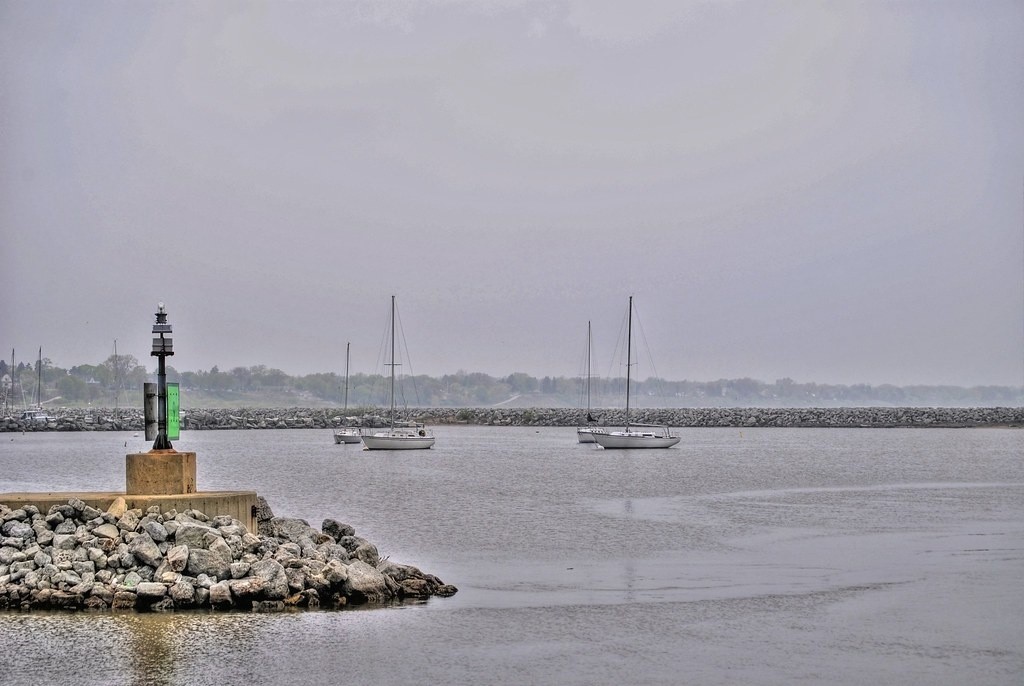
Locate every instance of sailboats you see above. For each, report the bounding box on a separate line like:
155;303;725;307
359;294;436;451
331;341;377;444
590;296;682;450
575;320;610;443
